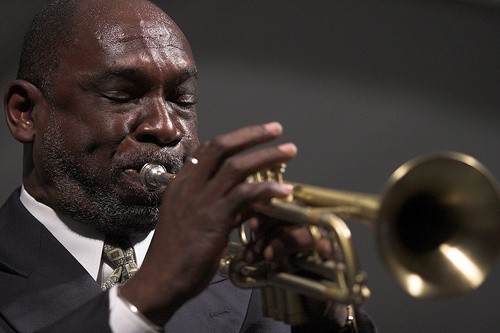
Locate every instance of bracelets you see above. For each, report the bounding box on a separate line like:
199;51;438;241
339;303;361;333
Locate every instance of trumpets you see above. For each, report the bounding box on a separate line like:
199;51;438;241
140;151;499;325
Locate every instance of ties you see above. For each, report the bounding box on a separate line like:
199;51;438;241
101;240;138;292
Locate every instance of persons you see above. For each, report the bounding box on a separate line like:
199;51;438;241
1;0;380;333
